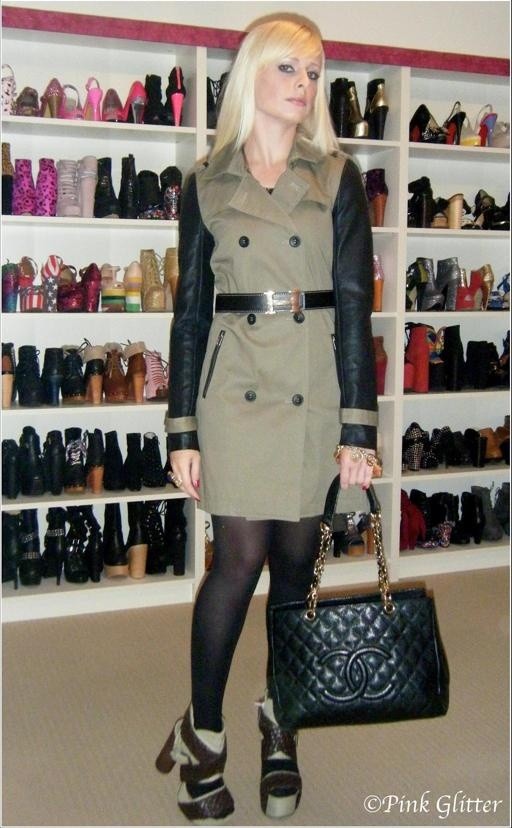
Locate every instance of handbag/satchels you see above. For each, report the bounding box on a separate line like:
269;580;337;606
270;469;450;731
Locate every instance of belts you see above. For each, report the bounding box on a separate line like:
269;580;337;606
213;288;340;316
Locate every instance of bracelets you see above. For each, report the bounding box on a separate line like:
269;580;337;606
333;443;378;467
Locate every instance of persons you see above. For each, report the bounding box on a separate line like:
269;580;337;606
154;12;380;827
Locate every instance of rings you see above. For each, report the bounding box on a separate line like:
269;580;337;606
166;470;182;488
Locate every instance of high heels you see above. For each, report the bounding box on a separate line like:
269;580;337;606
330;77;501;147
373;320;509;393
366;167;510;229
0;143;181;222
255;689;305;820
1;424;187;591
399;422;511;552
2;249;178;314
153;701;235;824
372;254;510;311
328;511;376;558
1;336;173;406
2;60;186;126
